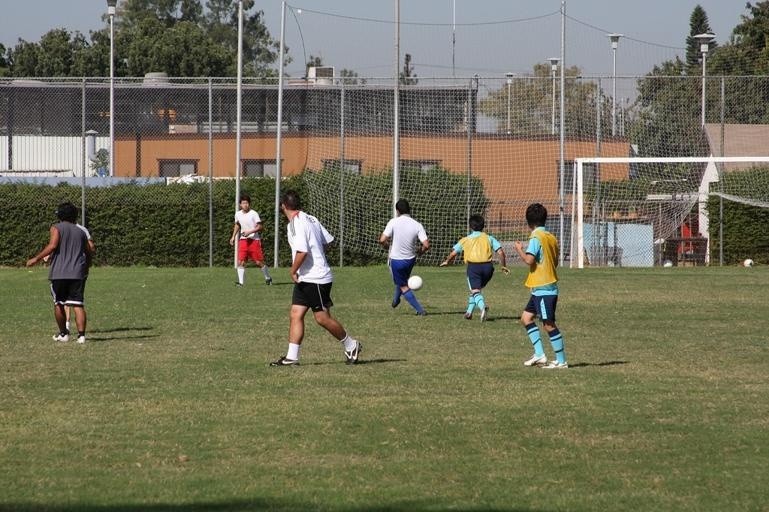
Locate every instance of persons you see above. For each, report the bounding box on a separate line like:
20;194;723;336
514;202;571;370
378;199;432;316
26;202;93;344
229;196;274;286
43;209;97;333
271;189;362;366
439;214;512;324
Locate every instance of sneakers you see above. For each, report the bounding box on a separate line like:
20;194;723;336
269;355;301;366
415;310;427;315
392;298;400;308
265;276;272;286
76;336;85;343
480;305;489;323
344;340;362;364
52;333;70;342
232;281;244;288
464;311;472;319
541;359;569;369
523;354;547;366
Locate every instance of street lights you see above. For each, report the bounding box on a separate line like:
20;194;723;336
502;73;517;139
603;32;626;139
544;57;561;138
691;32;717;133
105;1;119;173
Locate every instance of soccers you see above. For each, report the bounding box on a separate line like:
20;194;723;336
408;275;422;290
744;259;754;267
